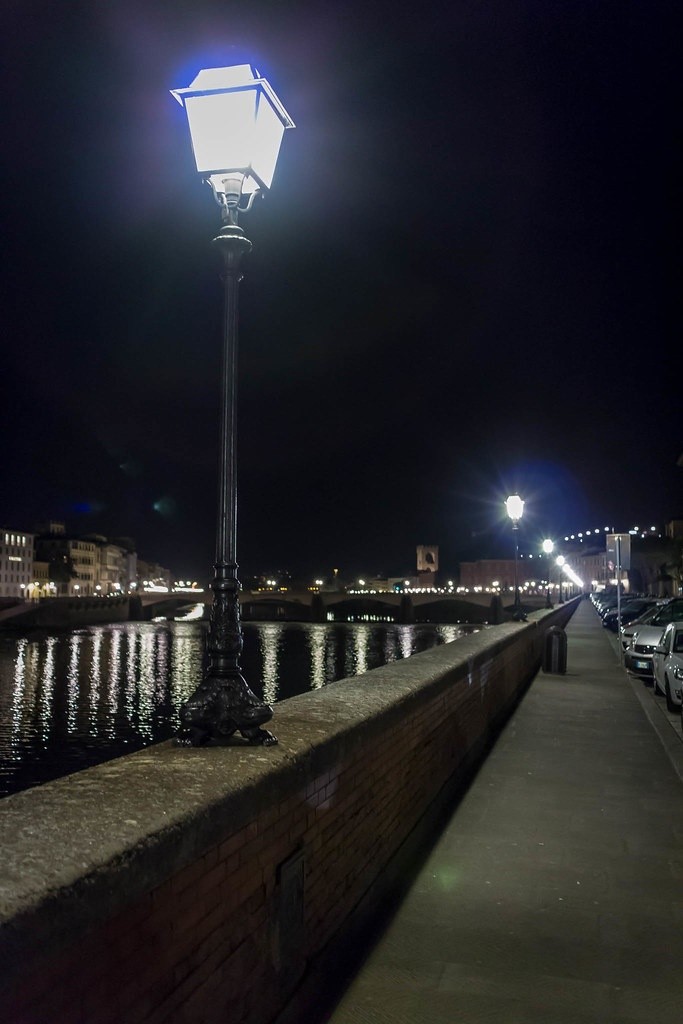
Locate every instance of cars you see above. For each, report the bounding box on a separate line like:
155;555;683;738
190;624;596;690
589;591;675;635
621;608;663;652
652;622;683;713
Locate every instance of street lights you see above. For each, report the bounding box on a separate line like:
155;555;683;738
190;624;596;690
541;538;555;609
167;62;296;749
505;494;526;621
558;555;584;604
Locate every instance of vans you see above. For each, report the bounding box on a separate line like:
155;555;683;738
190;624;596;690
623;597;683;681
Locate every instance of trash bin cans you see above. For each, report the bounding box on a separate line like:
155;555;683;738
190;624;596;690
541;626;568;675
